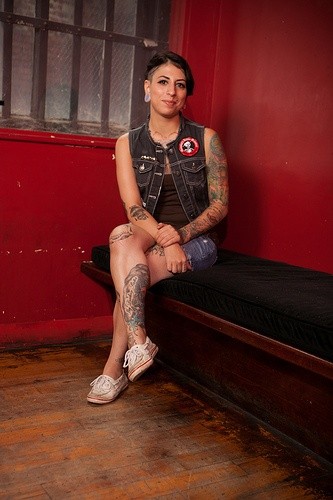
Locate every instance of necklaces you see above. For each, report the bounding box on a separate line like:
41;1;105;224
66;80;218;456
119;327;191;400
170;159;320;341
149;128;180;144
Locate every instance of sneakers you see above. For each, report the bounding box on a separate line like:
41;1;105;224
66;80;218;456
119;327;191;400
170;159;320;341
87;369;128;404
123;335;159;383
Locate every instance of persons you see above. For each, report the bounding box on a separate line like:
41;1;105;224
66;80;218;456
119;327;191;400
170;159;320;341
83;51;230;406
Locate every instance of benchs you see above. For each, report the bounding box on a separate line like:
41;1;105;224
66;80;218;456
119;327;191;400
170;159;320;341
81;241;333;470
